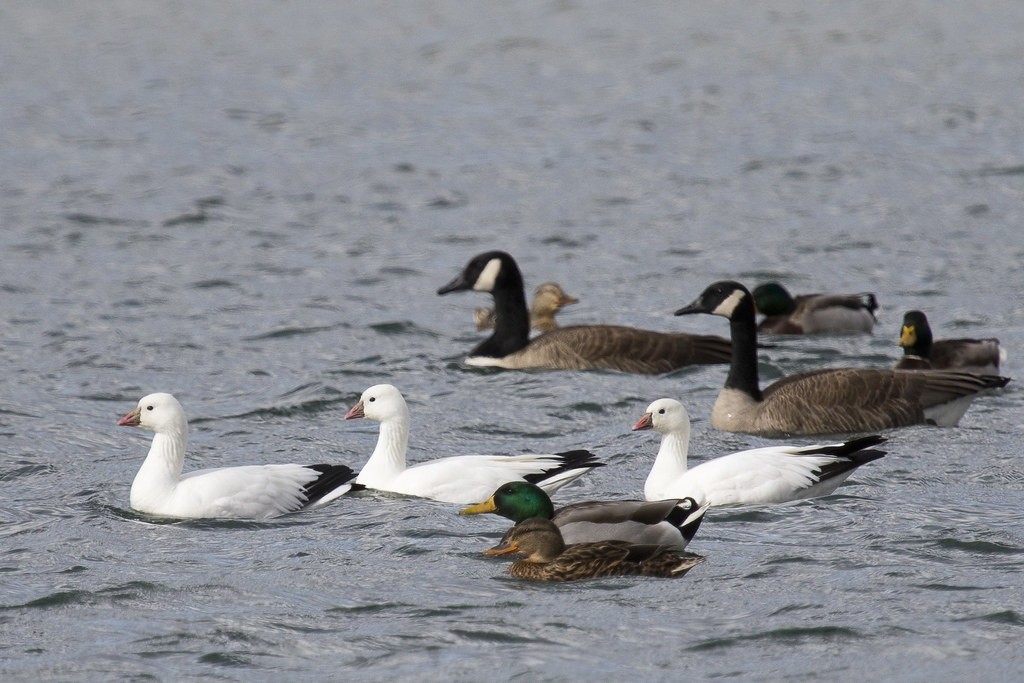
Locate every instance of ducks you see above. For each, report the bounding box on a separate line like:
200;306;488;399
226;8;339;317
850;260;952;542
482;516;711;583
747;283;880;335
892;310;1007;429
460;480;712;552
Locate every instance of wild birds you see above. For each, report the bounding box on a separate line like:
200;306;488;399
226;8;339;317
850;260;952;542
435;251;733;377
116;393;369;519
473;282;580;337
631;398;889;510
344;384;608;504
672;281;1017;440
